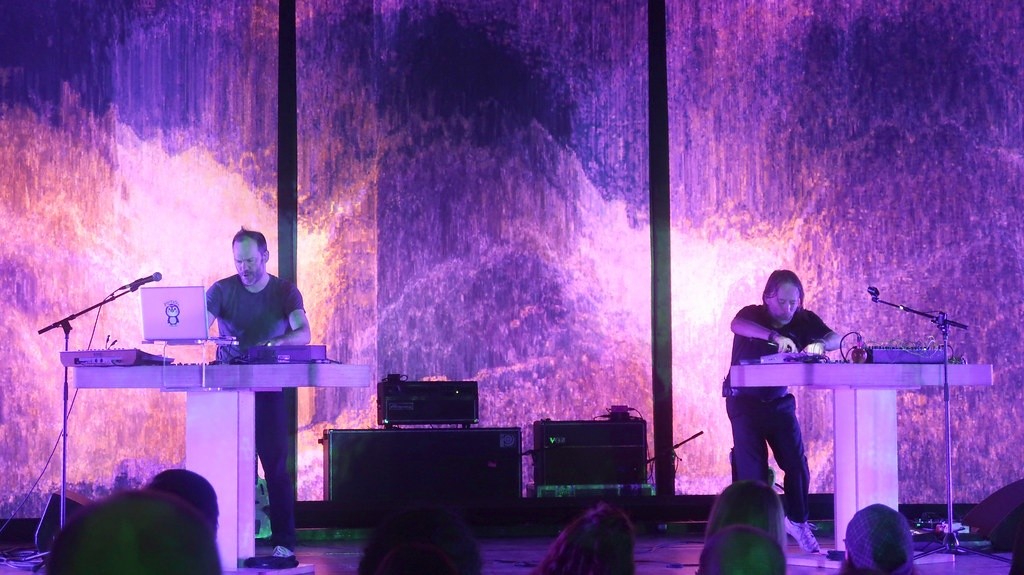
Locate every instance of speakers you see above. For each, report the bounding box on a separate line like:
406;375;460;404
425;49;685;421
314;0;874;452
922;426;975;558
319;428;522;503
35;490;97;558
532;421;648;487
961;477;1024;553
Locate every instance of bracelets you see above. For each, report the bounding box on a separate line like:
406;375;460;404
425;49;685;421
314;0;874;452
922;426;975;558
815;338;827;352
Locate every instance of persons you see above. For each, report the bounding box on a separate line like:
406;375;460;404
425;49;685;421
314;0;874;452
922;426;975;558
44;468;1024;575
722;270;843;555
205;226;311;558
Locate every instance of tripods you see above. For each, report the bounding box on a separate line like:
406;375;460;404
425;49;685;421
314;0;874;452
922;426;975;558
865;284;1012;564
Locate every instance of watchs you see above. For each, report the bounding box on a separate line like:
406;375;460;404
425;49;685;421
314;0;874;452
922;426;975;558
769;330;776;343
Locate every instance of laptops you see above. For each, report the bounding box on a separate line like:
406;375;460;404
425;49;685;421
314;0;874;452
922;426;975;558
140;286;237;341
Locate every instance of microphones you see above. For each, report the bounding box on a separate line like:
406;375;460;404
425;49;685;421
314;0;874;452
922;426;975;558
117;272;162;290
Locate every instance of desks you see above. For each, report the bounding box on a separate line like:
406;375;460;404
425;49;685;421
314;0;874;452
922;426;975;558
72;363;374;575
731;362;993;570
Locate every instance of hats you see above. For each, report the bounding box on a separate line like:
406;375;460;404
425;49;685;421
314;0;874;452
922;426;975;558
846;504;914;575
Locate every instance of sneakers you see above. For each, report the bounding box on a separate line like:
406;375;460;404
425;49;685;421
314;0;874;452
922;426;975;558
272;546;293;557
785;516;820;553
